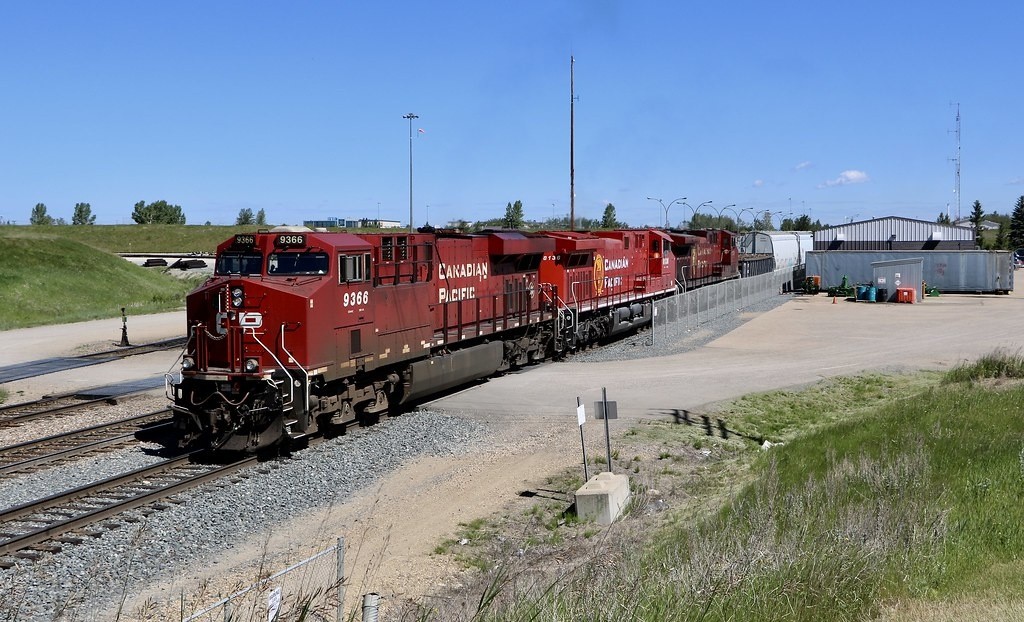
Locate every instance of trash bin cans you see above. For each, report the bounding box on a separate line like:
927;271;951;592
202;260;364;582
896;288;915;304
866;287;875;300
857;286;866;299
878;287;888;301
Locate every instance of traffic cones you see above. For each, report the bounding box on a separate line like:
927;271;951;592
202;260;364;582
832;296;837;305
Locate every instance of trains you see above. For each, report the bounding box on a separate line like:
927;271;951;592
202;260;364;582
165;226;814;452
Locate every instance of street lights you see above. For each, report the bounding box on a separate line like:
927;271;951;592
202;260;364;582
647;197;803;229
403;112;419;233
